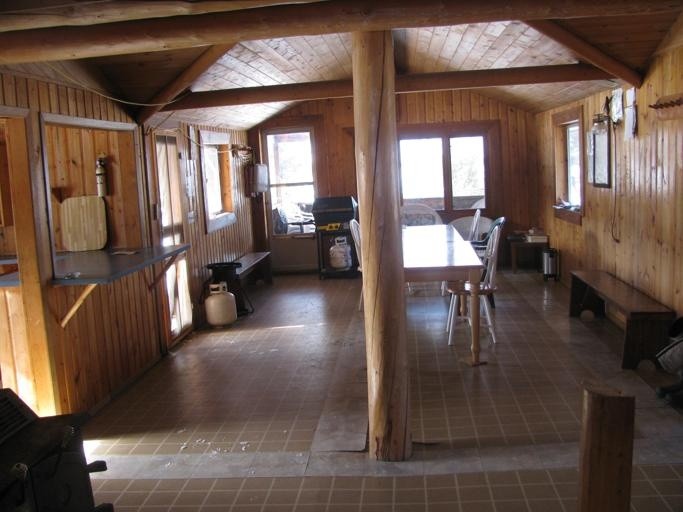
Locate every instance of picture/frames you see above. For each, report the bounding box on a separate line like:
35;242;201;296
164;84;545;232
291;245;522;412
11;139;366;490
592;117;610;188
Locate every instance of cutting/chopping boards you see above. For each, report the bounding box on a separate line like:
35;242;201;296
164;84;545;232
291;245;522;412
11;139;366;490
59;196;107;251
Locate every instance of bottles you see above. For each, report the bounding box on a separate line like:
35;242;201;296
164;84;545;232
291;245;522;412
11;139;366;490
447;223;454;241
95;159;106;198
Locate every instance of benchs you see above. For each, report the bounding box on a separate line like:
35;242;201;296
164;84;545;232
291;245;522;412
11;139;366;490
566;270;671;369
235;251;273;313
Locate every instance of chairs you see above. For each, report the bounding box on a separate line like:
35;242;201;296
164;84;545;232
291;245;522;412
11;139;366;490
345;204;506;343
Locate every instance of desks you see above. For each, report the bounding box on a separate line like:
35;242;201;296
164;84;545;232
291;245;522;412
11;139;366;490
509;233;547;272
